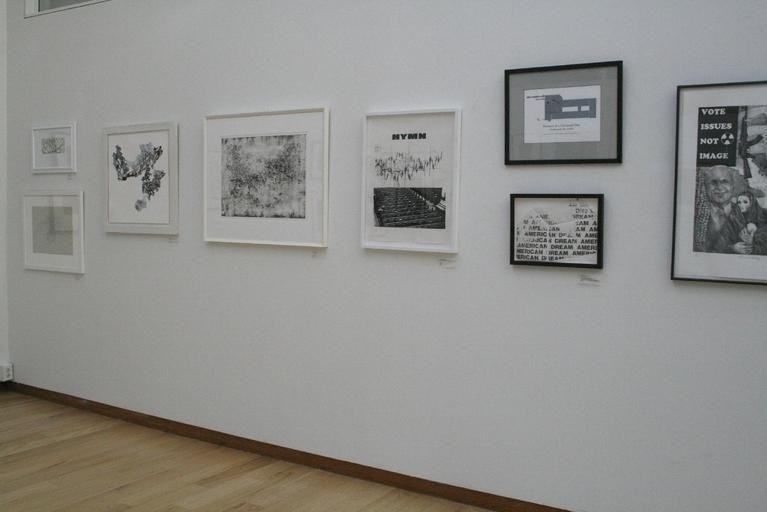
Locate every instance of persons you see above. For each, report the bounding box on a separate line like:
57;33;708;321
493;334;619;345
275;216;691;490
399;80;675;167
693;164;754;254
735;191;767;256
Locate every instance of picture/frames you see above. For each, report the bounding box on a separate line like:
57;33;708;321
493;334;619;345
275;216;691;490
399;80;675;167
360;107;461;253
671;80;767;285
103;122;179;235
505;61;622;164
24;191;84;274
510;194;603;268
203;106;329;247
30;121;77;173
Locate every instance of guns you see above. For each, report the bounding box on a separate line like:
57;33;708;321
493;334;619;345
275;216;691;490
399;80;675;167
738;107;762;179
